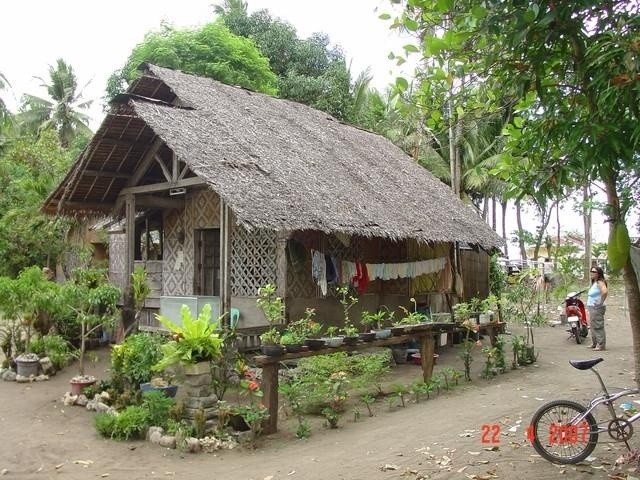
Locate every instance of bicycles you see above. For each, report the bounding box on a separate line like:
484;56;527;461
528;356;639;469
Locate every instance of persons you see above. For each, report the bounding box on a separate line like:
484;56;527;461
542;258;554;298
586;265;610;352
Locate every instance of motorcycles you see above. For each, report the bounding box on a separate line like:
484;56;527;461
561;289;589;345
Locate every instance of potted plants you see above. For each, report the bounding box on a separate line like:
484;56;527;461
0;264;66;377
150;304;228;374
59;267;123;396
257;283;502;356
512;268;544;363
141;376;178;399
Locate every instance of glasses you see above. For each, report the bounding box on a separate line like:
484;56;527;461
590;271;597;273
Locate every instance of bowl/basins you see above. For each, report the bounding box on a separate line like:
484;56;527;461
411;352;441;365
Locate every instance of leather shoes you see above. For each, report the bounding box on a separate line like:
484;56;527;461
592;347;602;351
587;345;595;348
